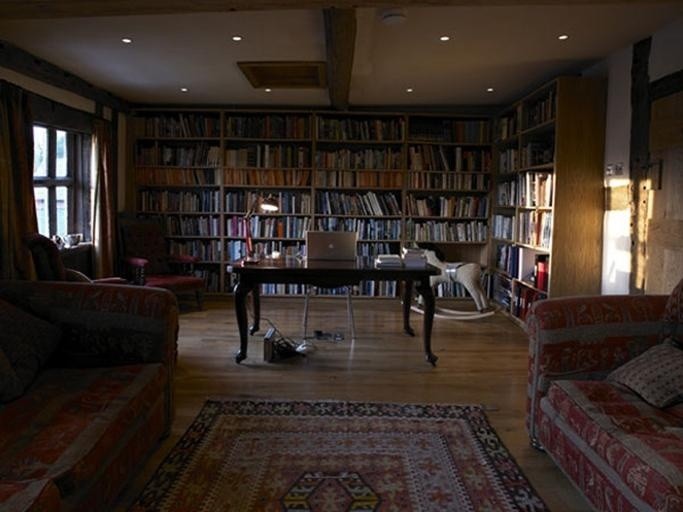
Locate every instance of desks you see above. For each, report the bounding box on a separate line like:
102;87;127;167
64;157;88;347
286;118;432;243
225;255;441;367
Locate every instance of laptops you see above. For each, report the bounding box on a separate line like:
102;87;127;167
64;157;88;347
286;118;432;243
306;230;358;262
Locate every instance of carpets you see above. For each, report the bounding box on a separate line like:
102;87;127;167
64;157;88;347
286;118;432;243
129;397;551;511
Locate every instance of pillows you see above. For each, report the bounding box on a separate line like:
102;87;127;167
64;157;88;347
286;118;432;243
0;298;63;401
605;342;681;408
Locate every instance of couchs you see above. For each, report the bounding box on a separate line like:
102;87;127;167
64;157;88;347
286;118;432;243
523;274;680;511
1;279;181;512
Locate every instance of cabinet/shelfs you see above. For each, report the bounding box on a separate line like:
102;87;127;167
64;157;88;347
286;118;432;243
486;77;606;331
128;107;486;299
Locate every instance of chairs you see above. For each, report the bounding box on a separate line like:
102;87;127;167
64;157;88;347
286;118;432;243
303;231;356;331
22;232;126;287
114;210;204;310
